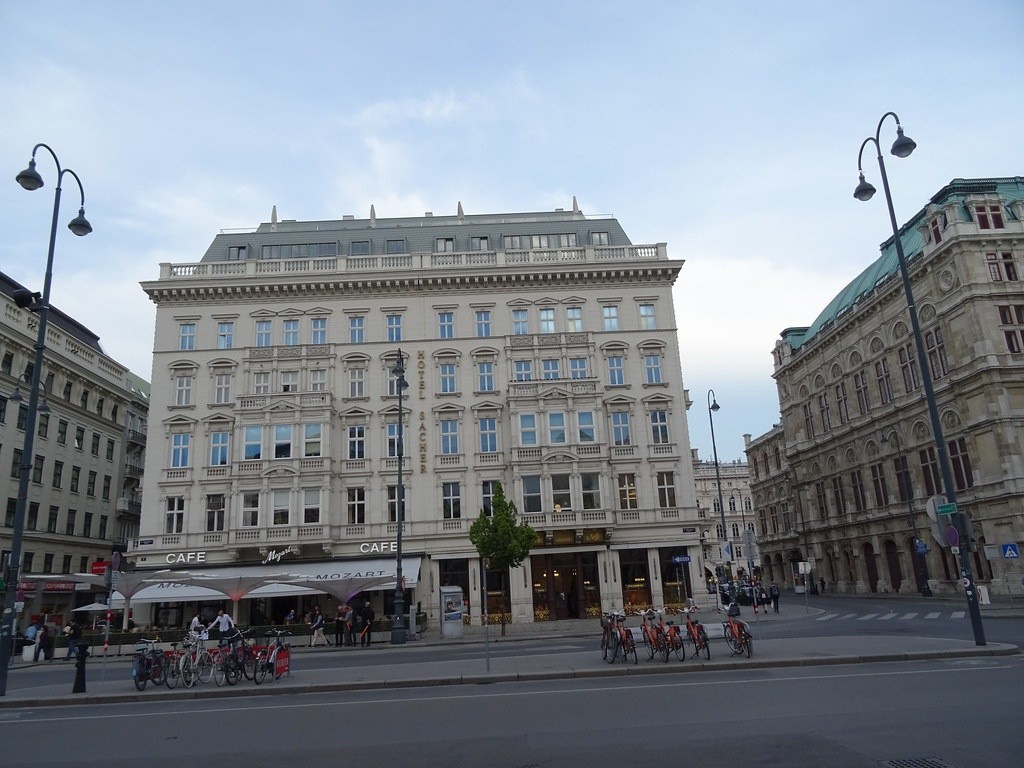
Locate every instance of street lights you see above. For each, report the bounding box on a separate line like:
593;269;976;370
708;388;737;598
852;108;988;647
389;347;410;642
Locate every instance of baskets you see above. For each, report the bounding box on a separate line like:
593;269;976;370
601;618;610;627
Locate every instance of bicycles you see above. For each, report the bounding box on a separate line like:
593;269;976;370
713;601;753;659
670;606;711;660
649;605;686;663
131;627;293;689
773;598;779;613
598;608;668;663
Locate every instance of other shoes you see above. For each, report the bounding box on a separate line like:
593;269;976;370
335;645;338;647
361;645;364;647
340;645;342;647
366;644;370;647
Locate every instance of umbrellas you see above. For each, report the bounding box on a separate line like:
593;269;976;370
71;602;109;629
457;201;465;225
573;195;579;214
272;205;277;232
370;204;376;228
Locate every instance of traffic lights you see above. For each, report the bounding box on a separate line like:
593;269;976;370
1;142;94;697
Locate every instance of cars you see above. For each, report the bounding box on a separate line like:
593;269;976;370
708;582;771;606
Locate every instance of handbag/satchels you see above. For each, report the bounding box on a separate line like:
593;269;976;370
771;599;774;608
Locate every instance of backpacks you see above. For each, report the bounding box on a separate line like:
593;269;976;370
760;592;766;598
772;586;778;594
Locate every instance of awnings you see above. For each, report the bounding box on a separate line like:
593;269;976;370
107;555;422;605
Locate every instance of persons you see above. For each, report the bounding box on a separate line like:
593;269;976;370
190;613;201;632
820;577;826;593
750;581;780;613
286;610;296;625
207;609;235;648
25;620;82;663
305;601;374;647
